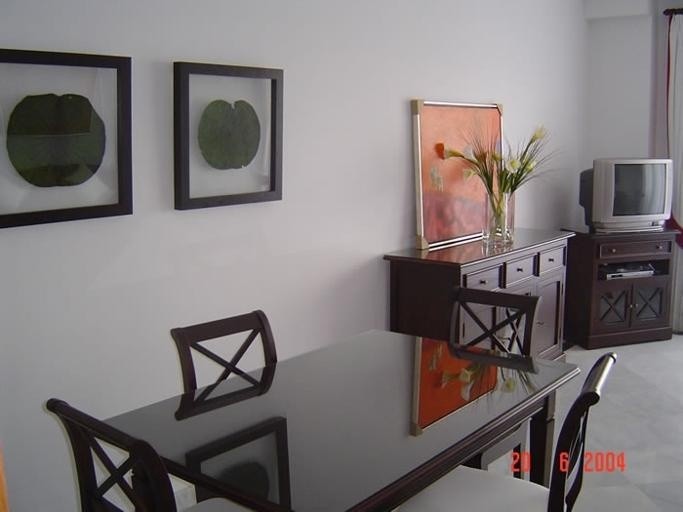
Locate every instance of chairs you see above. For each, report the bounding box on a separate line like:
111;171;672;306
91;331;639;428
170;309;277;392
393;352;618;512
175;363;276;420
46;398;253;512
451;285;542;479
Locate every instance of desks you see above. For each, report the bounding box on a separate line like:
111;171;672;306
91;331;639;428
104;327;579;512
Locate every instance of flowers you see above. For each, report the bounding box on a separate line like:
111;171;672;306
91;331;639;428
440;359;536;411
436;122;558;238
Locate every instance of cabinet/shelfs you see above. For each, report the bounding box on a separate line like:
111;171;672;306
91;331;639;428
383;228;576;363
559;229;681;349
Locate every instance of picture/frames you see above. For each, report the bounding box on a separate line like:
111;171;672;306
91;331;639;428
0;48;133;229
173;62;284;211
185;414;292;509
412;99;503;250
412;337;500;436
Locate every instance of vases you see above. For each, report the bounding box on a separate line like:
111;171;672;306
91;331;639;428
484;192;513;245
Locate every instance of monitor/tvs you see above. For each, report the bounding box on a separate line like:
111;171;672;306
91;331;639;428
579;158;673;232
